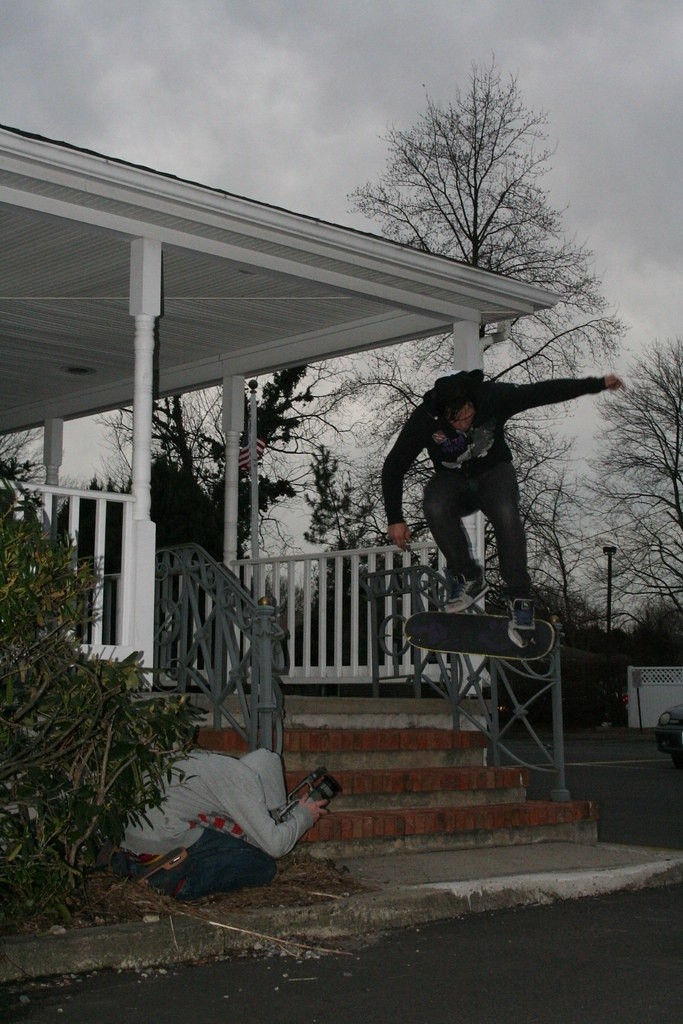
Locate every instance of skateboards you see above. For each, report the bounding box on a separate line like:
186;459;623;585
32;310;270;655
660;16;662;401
402;609;558;662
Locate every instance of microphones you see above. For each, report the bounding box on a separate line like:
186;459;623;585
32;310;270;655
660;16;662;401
312;767;328;780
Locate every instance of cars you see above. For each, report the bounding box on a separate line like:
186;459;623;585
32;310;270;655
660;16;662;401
653;702;683;769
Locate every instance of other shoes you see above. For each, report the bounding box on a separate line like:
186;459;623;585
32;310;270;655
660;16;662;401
136;846;190;886
98;843;130;883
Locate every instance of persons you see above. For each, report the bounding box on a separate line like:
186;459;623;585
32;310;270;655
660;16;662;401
120;748;331;904
380;368;626;650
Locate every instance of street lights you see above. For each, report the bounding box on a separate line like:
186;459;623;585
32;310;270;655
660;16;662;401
601;545;618;723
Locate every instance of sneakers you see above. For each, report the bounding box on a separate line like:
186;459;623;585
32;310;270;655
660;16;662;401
505;596;536;648
445;564;490;613
